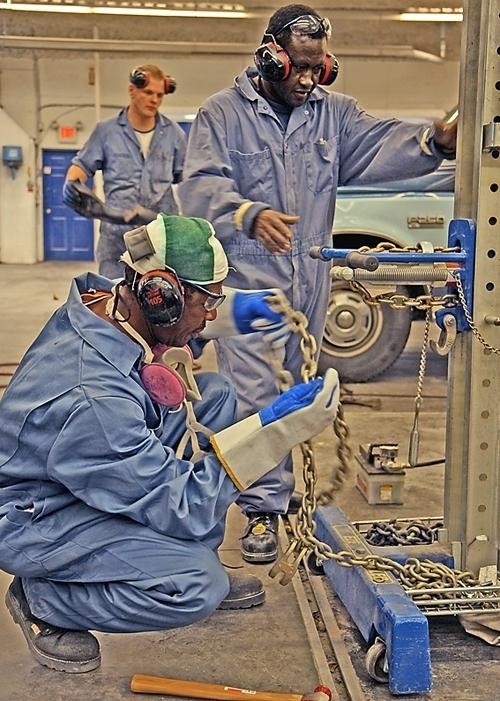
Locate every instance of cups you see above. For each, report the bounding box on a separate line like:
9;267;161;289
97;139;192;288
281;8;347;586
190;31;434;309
177;277;226;312
275;14;331;41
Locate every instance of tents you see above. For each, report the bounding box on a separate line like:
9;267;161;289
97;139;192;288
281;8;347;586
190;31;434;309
250;26;341;84
119;225;186;328
128;65;179;94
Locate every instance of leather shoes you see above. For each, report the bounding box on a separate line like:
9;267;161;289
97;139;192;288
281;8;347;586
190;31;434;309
200;289;288;341
61;179;82;209
211;367;339;492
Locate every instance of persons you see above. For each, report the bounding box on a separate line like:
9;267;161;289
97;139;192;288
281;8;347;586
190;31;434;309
61;63;186;282
1;212;340;674
182;4;459;564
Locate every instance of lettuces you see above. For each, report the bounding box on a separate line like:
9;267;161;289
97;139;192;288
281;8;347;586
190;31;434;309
128;673;336;701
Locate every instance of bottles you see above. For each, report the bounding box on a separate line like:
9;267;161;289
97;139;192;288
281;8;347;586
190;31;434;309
122;216;229;285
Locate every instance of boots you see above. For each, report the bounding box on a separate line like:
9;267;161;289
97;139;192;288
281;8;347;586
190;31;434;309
315;162;456;383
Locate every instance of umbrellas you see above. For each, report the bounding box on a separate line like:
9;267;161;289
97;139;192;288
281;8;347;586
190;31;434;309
5;576;102;673
242;513;277;564
219;573;266;611
